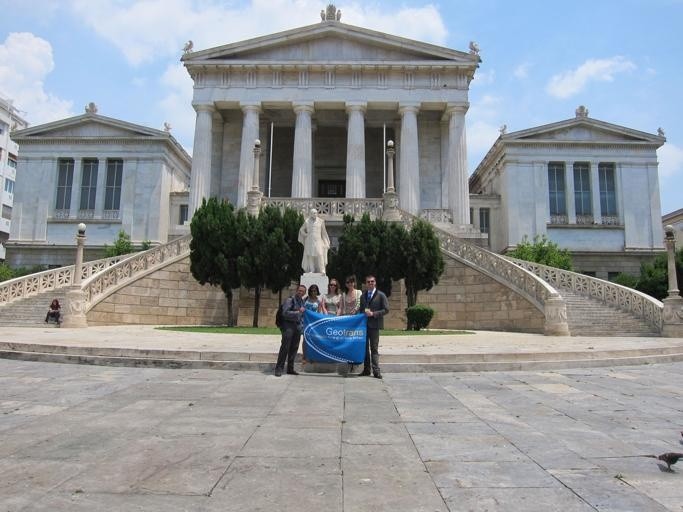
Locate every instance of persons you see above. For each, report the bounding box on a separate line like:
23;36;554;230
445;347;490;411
343;274;362;315
298;209;330;273
321;278;343;316
302;285;321;364
275;285;306;376
358;275;390;379
44;299;61;322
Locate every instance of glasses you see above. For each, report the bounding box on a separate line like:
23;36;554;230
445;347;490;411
345;282;353;284
368;280;375;283
330;284;336;287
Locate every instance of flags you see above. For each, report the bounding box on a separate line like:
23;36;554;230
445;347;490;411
304;306;368;365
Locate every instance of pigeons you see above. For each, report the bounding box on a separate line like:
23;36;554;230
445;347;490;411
656;451;683;472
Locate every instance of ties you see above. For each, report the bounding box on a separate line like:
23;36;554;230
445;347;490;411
367;292;372;304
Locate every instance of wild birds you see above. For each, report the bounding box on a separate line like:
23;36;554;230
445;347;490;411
500;124;507;134
320;9;325;20
656;127;665;136
336;9;342;21
163;121;172;131
468;40;480;53
181;40;193;54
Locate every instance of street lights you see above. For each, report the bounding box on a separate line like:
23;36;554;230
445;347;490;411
385;139;394;189
73;223;88;286
251;139;262;188
663;224;678;291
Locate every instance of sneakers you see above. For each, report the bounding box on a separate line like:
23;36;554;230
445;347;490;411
275;368;299;376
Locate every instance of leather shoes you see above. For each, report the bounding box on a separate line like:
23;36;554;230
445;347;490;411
359;371;382;379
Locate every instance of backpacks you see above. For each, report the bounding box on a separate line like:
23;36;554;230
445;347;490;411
276;296;294;328
